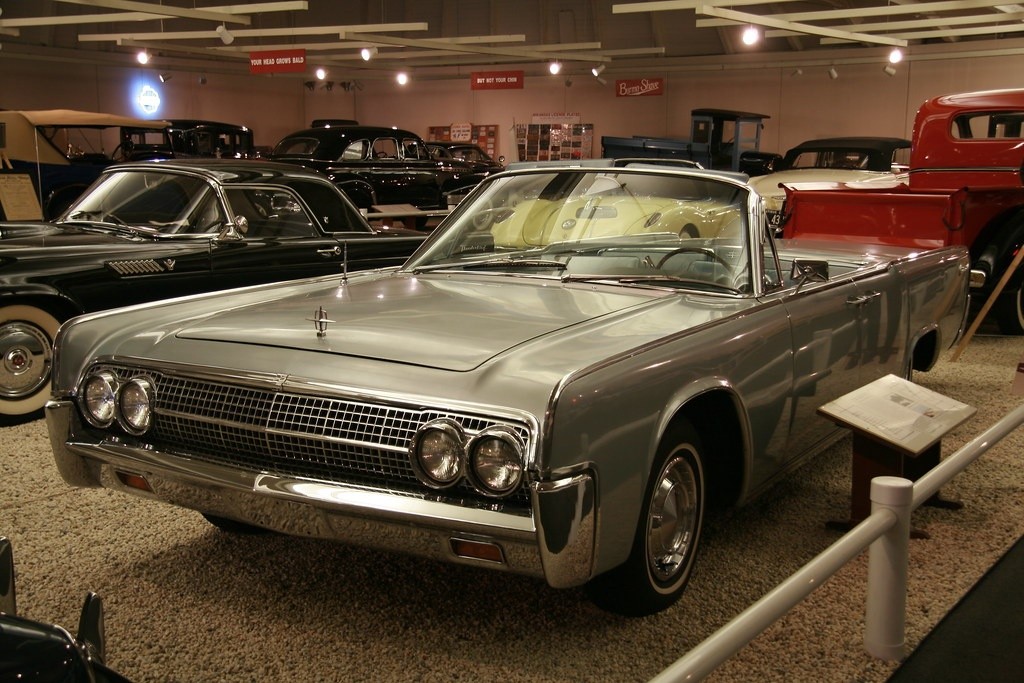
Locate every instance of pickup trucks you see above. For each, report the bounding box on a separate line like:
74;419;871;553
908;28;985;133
773;87;1024;335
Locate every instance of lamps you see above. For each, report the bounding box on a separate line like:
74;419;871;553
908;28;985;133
550;59;563;74
883;62;897;77
216;22;234;45
827;65;838;81
362;46;379;62
597;77;607;86
592;63;606;77
159;66;173;83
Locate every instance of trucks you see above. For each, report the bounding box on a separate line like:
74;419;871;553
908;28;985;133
600;107;786;180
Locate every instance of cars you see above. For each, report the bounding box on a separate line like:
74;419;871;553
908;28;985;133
747;136;912;237
44;158;972;621
491;186;751;251
1;109;507;229
0;158;494;427
